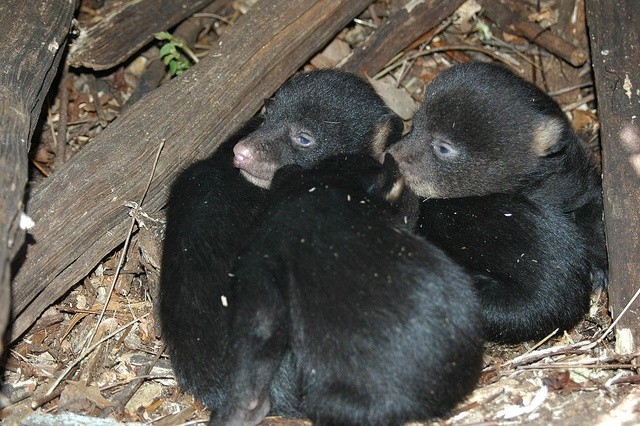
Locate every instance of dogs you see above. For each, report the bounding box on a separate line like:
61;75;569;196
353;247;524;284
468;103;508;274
209;164;487;426
156;66;404;421
379;60;608;346
